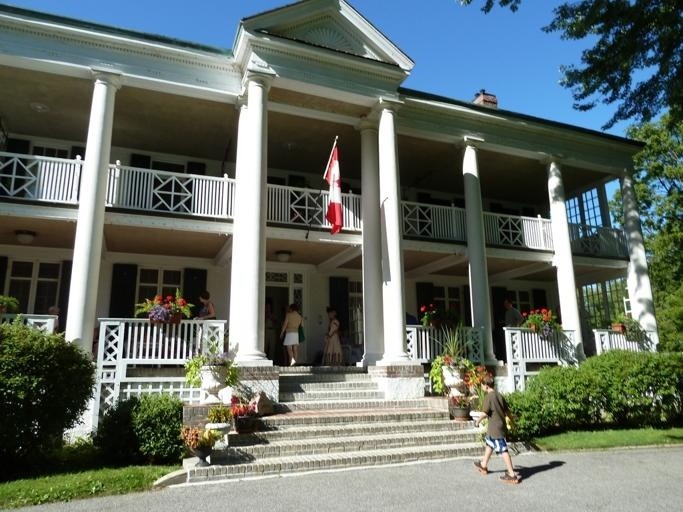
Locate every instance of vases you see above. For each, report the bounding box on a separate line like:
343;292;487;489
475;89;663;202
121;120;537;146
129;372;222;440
448;404;469;420
148;312;181;326
468;410;488;427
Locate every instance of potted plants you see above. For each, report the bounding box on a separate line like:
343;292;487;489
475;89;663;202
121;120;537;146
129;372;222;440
175;334;257;469
609;309;646;342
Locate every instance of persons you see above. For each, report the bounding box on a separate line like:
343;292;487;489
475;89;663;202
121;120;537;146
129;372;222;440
473;370;520;484
326;310;344;366
556;304;562;325
280;303;303;366
196;291;216;319
47;306;61;316
503;298;525;328
265;298;277;360
405;311;417;325
93;324;118;361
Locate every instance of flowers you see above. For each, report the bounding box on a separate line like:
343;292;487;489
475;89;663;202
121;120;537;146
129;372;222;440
519;307;563;339
418;301;495;410
131;283;198;326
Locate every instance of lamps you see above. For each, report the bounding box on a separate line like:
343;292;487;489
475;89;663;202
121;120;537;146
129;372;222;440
274;250;292;262
15;230;36;246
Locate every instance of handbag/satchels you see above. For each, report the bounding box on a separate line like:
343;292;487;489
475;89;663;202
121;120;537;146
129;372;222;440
299;327;304;342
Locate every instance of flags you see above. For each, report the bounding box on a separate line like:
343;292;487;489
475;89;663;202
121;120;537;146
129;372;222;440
324;142;343;234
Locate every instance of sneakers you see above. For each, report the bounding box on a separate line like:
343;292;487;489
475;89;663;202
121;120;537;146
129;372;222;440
500;474;518;484
474;462;487;474
290;359;296;366
